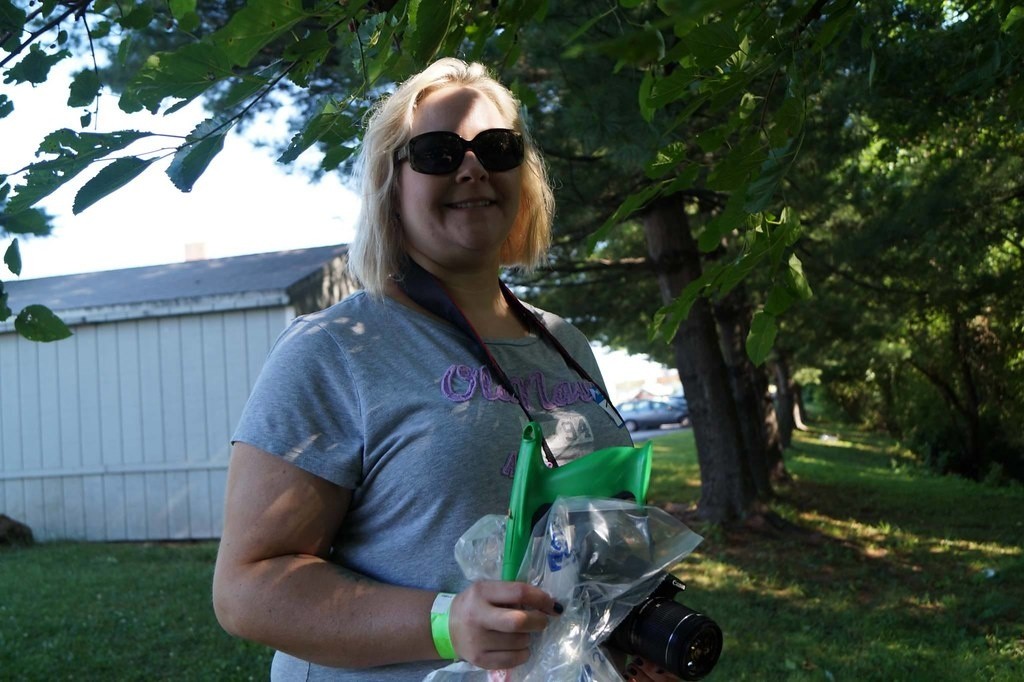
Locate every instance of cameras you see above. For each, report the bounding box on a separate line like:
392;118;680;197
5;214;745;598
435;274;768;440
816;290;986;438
567;534;728;682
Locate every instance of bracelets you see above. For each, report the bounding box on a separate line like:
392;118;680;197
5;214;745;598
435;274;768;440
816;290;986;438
430;592;457;660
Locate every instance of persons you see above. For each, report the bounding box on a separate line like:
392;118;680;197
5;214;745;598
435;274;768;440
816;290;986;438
213;59;635;682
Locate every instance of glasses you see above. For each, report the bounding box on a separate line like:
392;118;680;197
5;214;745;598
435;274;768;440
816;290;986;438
394;128;524;176
